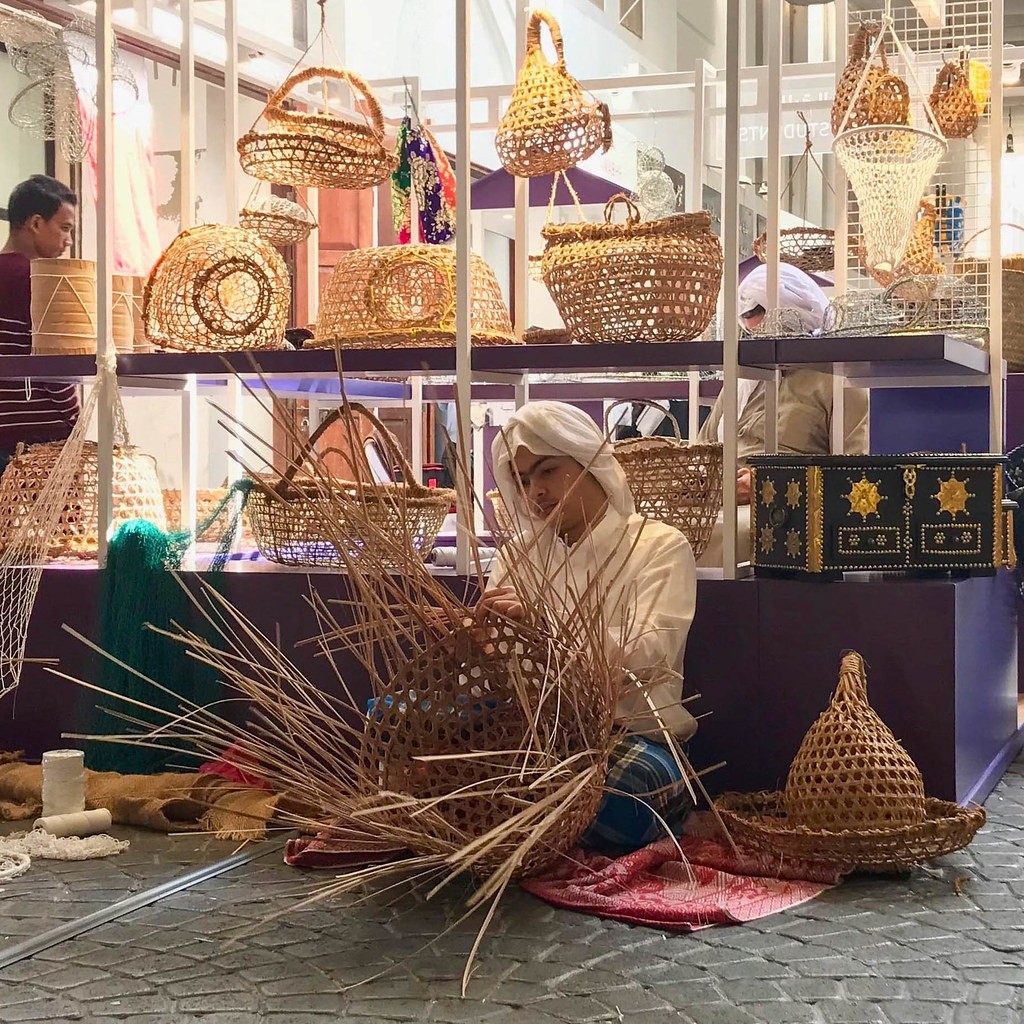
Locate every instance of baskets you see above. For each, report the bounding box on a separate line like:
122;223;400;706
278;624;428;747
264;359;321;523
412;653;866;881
241;180;320;244
142;221;291;350
542;191;724;340
746;21;1024;373
1;440;169;559
714;648;987;867
244;402;458;570
236;67;400;190
494;11;612;177
299;244;523;345
604;398;724;561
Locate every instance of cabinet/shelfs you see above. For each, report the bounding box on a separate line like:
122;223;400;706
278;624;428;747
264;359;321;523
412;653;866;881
0;0;1024;815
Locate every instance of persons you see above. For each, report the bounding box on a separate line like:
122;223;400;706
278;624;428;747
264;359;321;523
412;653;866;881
361;401;699;852
638;259;869;569
0;173;85;543
